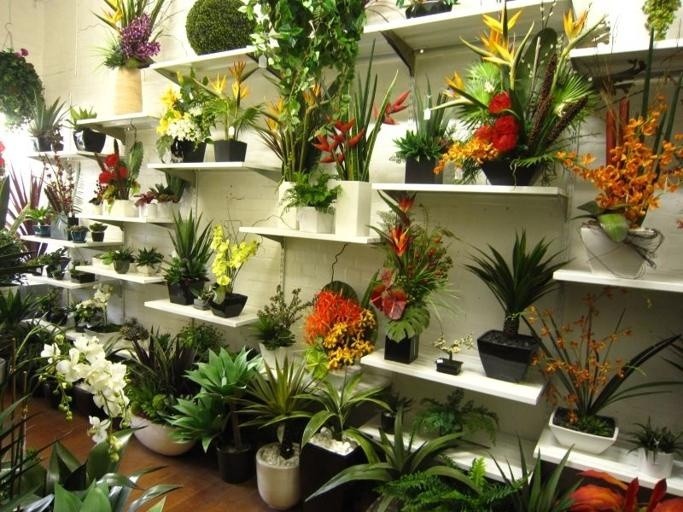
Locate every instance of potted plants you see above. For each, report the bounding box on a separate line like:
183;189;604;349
26;92;65;151
